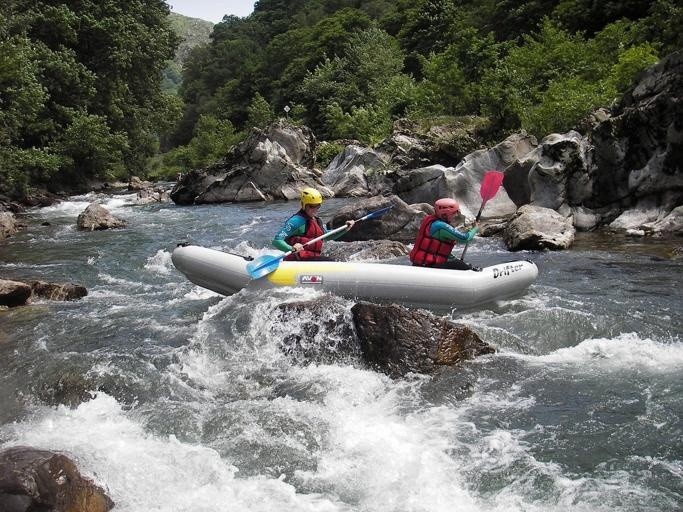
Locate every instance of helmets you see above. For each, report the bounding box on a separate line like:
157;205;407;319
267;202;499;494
301;188;322;210
435;198;459;221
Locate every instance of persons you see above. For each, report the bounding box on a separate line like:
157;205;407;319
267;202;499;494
409;198;480;269
271;188;354;261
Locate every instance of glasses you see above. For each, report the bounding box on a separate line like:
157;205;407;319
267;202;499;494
308;204;321;210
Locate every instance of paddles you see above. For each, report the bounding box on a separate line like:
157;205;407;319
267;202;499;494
460;171;504;262
247;204;395;281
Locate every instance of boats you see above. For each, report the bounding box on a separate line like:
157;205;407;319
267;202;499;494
171;245;539;309
